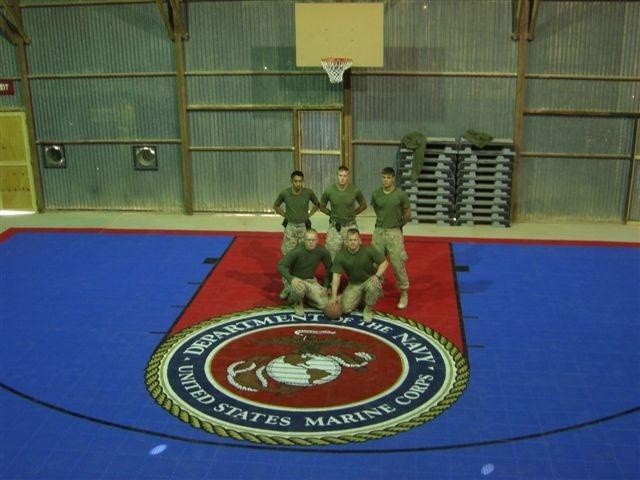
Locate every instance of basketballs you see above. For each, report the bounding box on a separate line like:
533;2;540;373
325;303;342;319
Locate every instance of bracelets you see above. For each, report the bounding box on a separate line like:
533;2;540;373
376;275;382;280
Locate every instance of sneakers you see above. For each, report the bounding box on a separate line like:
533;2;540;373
363;307;373;323
397;294;408;309
294;301;304;316
279;287;291;300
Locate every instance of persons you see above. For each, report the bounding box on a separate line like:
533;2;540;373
328;228;390;325
320;164;369;296
369;166;412;311
272;170;320;301
276;228;332;317
